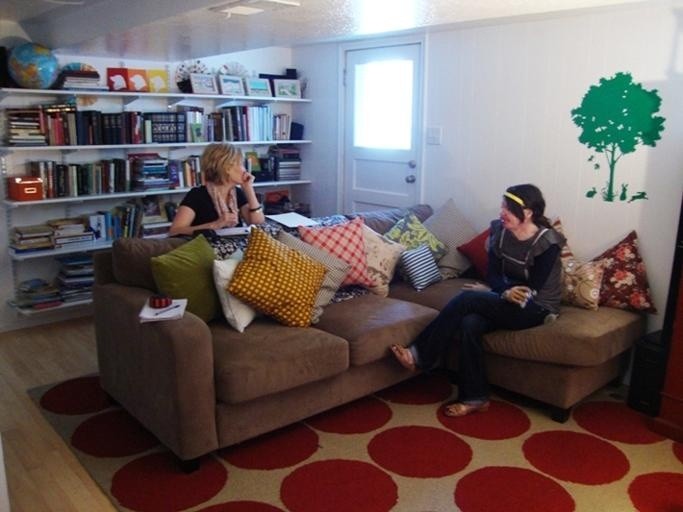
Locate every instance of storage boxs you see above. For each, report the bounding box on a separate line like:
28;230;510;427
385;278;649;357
5;176;44;202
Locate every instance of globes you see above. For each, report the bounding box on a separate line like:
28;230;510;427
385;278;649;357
7;43;59;89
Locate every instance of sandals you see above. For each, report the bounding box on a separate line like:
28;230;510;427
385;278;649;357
391;343;415;371
445;399;490;418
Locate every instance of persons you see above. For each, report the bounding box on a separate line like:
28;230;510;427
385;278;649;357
390;184;567;417
169;143;264;237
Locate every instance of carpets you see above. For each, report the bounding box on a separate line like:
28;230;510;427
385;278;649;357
27;371;683;512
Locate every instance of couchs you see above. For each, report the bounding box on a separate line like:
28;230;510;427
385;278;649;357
95;201;649;474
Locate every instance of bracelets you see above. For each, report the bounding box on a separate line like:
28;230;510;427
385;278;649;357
249;206;262;211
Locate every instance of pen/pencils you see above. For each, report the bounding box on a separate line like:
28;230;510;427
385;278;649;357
156;305;180;315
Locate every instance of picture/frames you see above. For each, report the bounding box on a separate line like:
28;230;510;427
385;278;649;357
245;77;273;97
273;78;302;98
219;74;245;96
189;72;219;94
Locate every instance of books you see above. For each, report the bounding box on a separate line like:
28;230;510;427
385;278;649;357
85;194;181;241
245;144;302;180
141;298;186;322
15;253;94;308
7;153;205;201
3;104;305;147
8;218;95;255
256;189;308;218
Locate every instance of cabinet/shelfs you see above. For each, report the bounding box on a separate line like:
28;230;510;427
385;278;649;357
0;86;314;317
